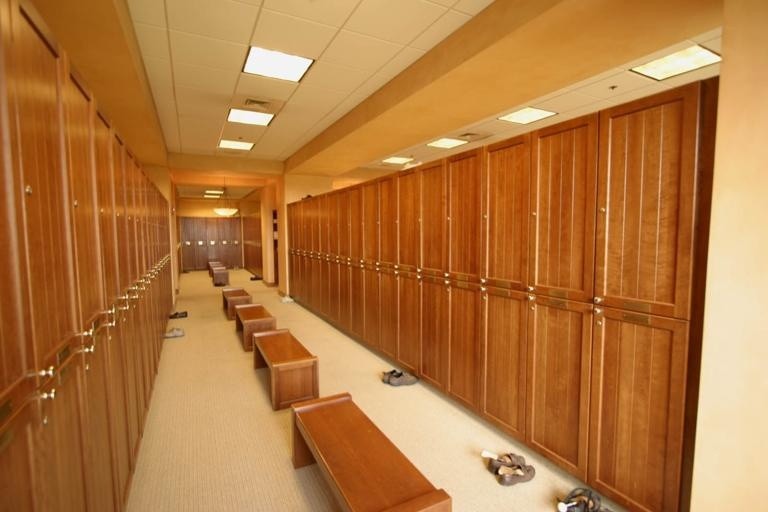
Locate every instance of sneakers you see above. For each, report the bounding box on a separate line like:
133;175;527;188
169;310;188;320
382;368;419;386
165;327;185;339
281;295;294;303
250;275;264;280
215;282;226;286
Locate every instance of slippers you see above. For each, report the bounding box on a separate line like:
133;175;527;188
558;485;614;512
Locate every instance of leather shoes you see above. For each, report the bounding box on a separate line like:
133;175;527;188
488;451;527;473
497;463;536;486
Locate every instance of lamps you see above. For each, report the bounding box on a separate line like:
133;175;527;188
214;138;258;153
226;107;278;129
240;43;318;86
200;189;228;201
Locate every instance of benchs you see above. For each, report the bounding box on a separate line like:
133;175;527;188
220;283;254;322
249;325;324;409
205;258;232;287
231;300;277;350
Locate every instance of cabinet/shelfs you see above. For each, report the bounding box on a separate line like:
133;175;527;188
175;209;248;272
4;5;185;506
286;76;717;512
